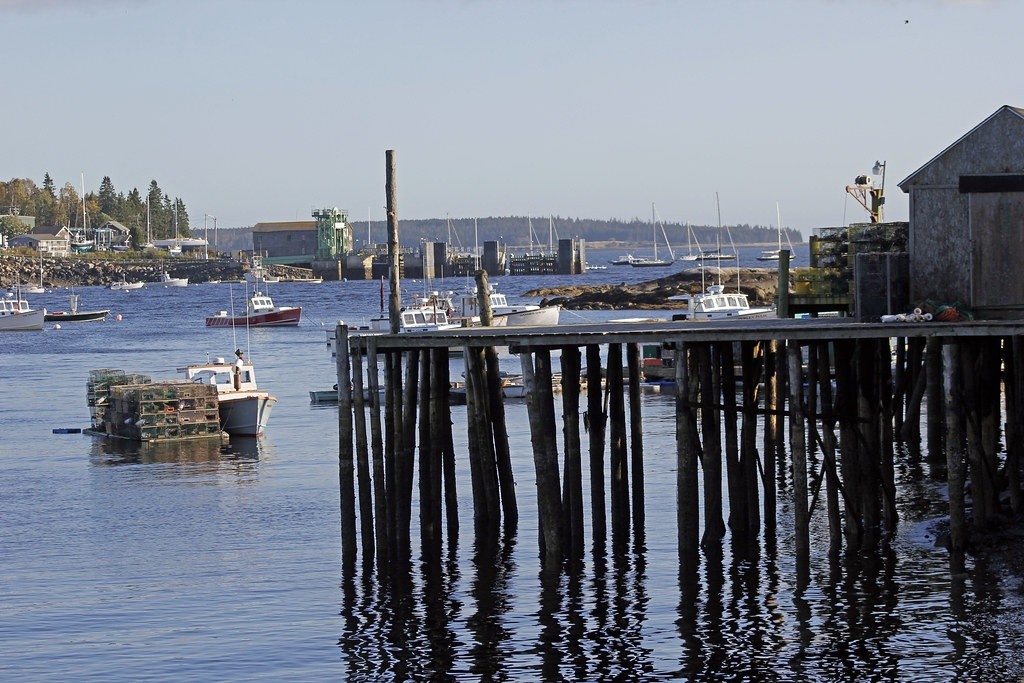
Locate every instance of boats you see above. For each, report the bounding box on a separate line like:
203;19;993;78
44;284;110;322
153;197;210;252
610;255;634;266
685;234;773;319
184;280;278;436
109;274;144;290
0;264;47;330
156;258;189;287
415;259;542;316
204;237;303;327
329;272;509;357
406;253;562;330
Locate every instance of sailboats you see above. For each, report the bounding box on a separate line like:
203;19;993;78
628;201;678;267
680;220;702;260
756;199;797;261
696;191;737;261
